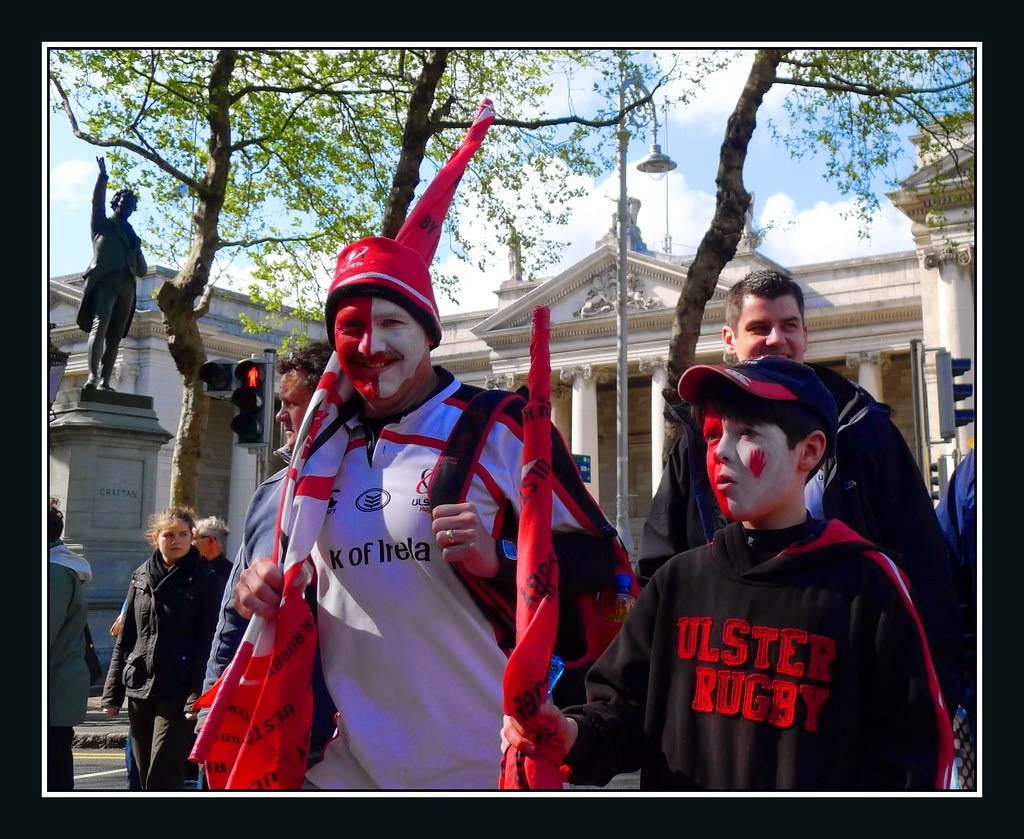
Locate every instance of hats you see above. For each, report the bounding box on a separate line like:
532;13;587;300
325;235;443;351
677;352;838;459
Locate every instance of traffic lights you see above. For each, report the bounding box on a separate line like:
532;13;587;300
930;458;947;502
230;358;269;447
198;358;241;399
935;351;974;438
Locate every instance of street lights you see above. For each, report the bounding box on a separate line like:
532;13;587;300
618;78;677;554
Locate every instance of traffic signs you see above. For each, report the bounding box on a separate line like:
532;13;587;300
568;455;591;483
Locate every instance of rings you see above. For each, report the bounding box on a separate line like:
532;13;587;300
446;529;455;546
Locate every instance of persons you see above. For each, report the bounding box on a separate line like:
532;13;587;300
46;495;93;792
196;338;335;793
76;157;147;393
935;434;977;568
634;269;966;728
234;236;639;789
499;354;962;792
101;507;234;791
